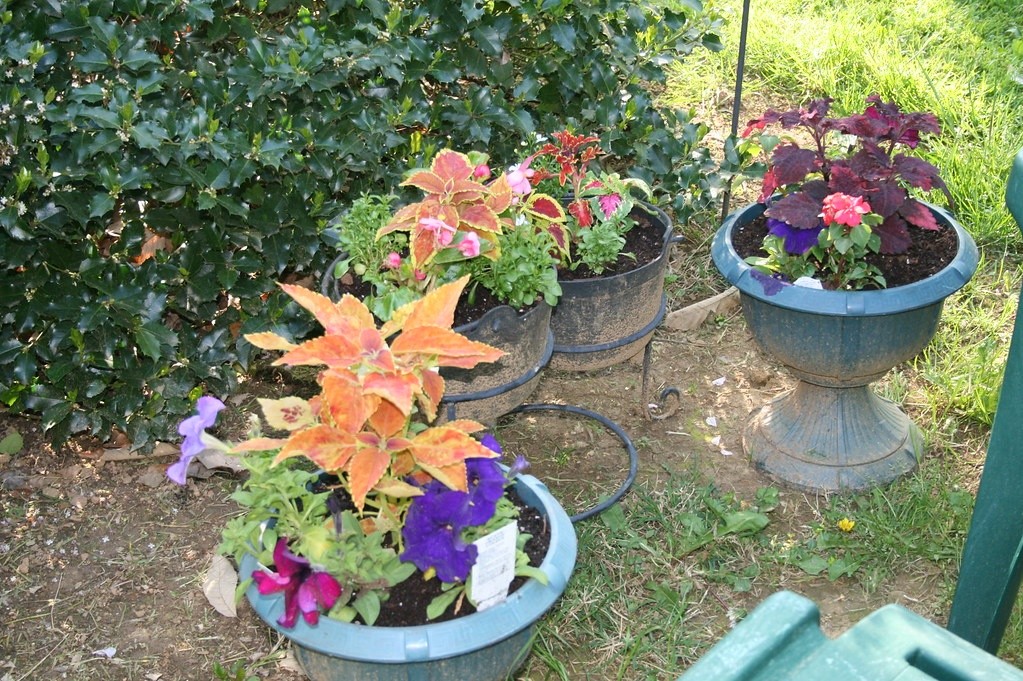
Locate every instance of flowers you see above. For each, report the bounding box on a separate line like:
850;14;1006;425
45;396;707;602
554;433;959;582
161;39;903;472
342;165;553;316
167;398;530;630
811;193;889;290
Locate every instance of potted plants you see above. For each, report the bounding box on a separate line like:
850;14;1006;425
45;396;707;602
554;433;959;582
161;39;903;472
503;129;673;369
323;147;570;427
214;275;578;680
709;88;977;495
